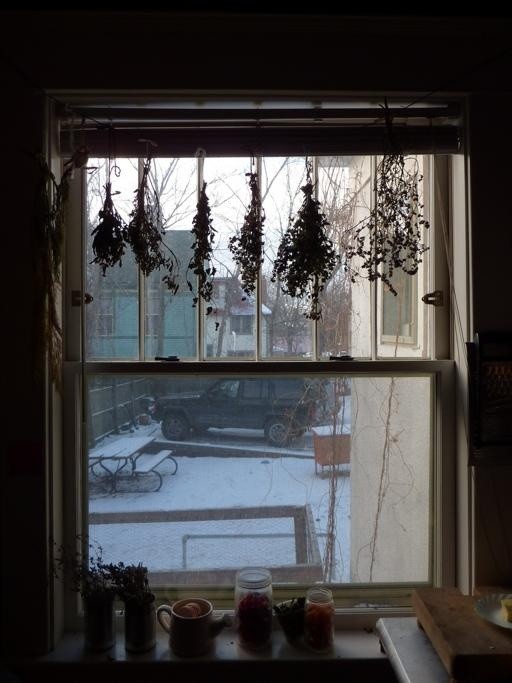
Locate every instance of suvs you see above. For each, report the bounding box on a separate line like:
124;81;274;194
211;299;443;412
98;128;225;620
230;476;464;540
151;378;340;447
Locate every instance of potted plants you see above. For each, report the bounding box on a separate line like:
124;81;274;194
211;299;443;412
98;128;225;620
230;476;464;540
46;532;115;652
97;560;158;654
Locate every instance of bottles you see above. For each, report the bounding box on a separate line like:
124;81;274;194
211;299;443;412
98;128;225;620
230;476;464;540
303;586;336;654
233;566;274;652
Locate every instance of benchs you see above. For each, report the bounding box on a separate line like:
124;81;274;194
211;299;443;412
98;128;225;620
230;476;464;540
133;449;178;492
88;460;101;487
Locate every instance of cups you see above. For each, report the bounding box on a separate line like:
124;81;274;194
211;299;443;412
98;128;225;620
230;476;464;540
124;593;155;653
80;594;115;651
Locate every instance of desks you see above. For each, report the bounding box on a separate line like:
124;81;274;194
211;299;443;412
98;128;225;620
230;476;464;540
311;423;351;479
89;436;155;494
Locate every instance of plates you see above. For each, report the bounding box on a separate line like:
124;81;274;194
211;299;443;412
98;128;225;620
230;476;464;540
473;592;512;630
